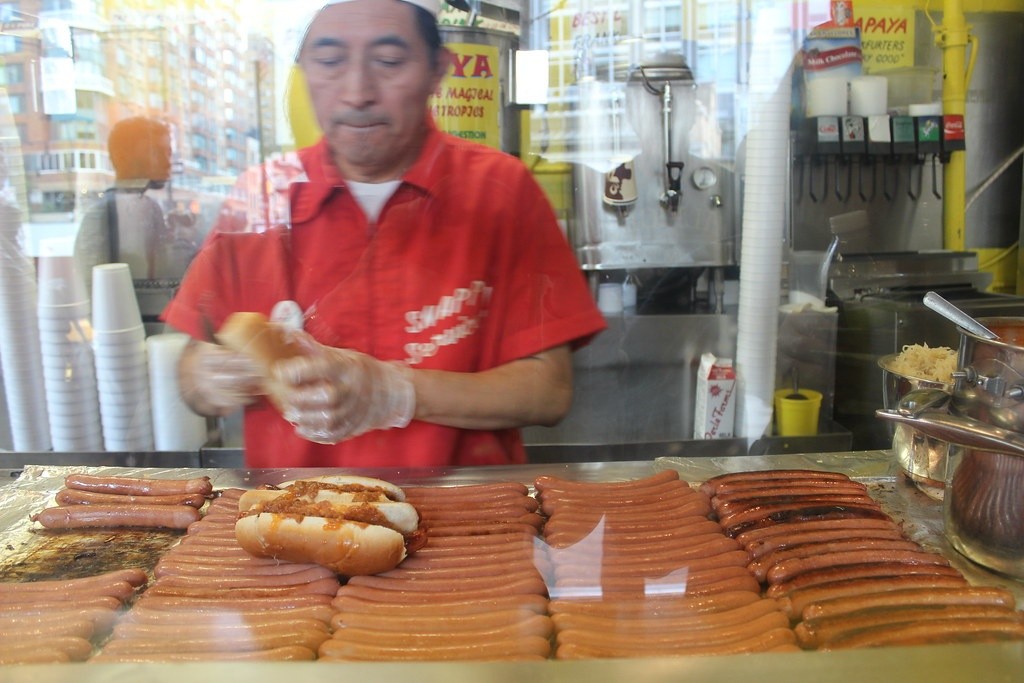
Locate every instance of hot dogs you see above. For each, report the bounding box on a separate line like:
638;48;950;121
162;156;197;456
235;474;428;576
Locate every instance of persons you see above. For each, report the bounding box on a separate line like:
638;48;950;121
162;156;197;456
71;117;176;281
599;55;742;316
158;0;608;472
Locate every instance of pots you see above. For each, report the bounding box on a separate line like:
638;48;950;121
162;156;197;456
876;317;1024;578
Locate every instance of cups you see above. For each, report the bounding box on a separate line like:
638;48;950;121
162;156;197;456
622;284;637;307
34;237;104;452
774;389;822;436
0;84;52;453
790;250;829;305
90;261;153;451
602;155;639;207
597;282;623;313
147;333;207;451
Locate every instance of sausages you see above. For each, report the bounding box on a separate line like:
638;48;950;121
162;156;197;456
315;481;555;665
29;473;212;532
0;570;149;666
534;467;1024;662
86;486;337;663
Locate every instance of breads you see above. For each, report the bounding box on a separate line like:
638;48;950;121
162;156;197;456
215;311;303;415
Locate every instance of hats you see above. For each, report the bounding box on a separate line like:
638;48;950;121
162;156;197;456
295;0;442;15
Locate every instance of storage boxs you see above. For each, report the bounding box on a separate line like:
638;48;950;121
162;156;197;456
692;351;736;439
